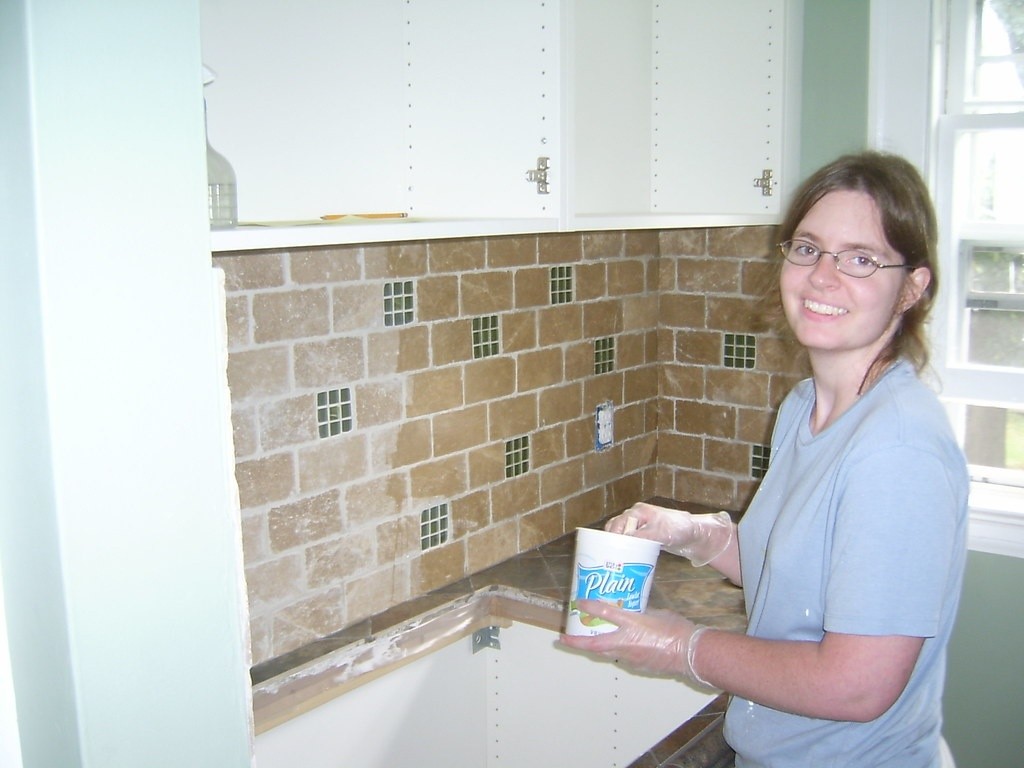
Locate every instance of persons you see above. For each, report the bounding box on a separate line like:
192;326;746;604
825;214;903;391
559;151;972;768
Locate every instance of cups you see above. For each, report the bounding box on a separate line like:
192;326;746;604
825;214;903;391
565;527;664;635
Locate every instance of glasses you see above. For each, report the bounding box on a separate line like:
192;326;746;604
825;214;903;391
777;239;919;280
